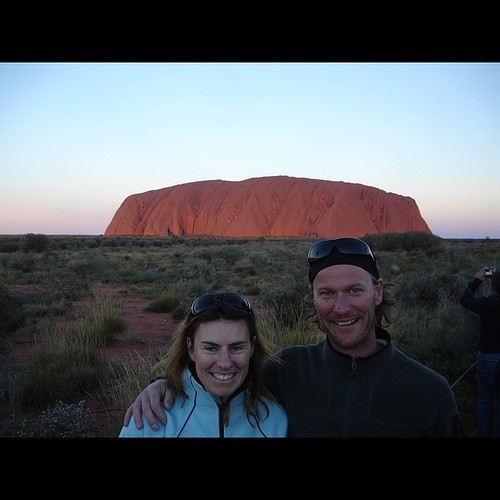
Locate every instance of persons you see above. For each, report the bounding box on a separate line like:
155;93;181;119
461;265;500;434
118;292;289;438
124;237;460;438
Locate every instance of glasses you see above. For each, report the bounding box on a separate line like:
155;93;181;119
189;292;252;322
307;237;376;264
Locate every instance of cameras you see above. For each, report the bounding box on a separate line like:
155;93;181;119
483;267;496;278
